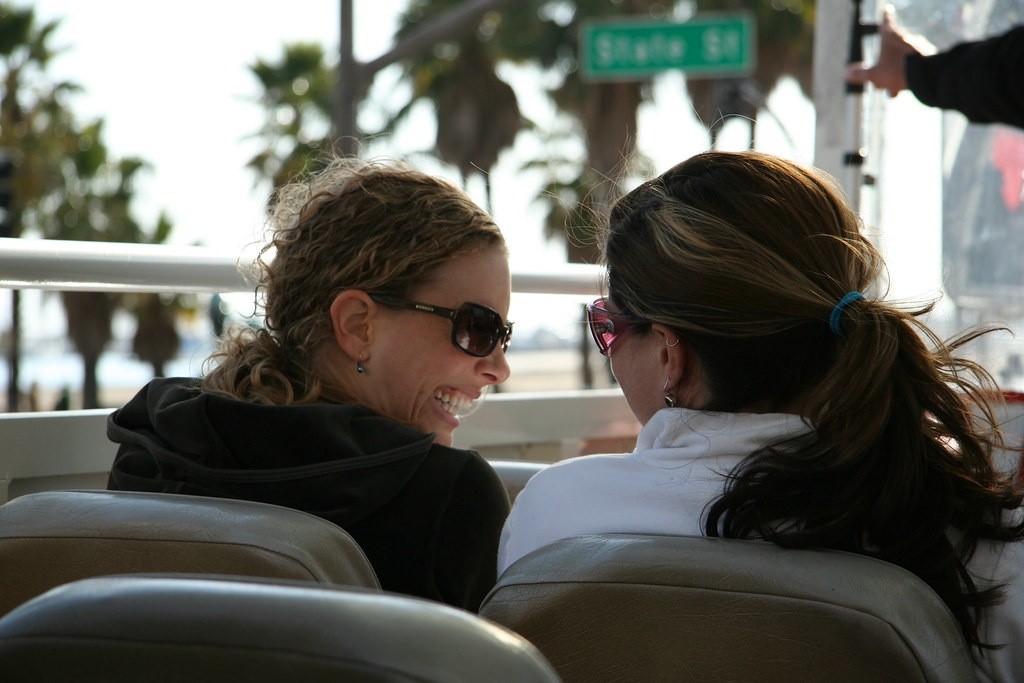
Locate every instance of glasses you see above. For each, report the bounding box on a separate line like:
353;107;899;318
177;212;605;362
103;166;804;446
585;297;655;357
368;292;513;358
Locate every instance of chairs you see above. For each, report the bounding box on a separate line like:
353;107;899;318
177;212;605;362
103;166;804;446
0;488;977;683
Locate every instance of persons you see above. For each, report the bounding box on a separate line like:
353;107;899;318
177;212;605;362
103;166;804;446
494;152;1024;683
841;2;1024;132
107;156;512;616
